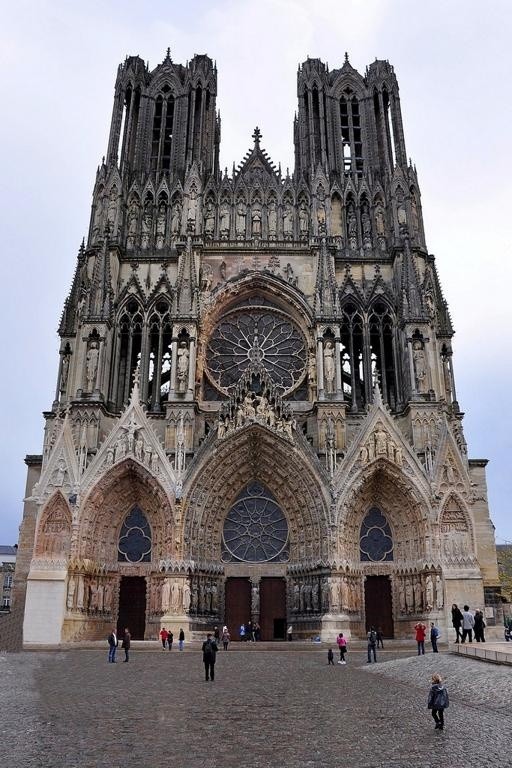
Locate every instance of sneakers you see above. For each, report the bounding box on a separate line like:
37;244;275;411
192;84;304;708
435;722;441;729
338;661;347;665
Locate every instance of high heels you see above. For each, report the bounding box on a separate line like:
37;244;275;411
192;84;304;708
455;641;459;643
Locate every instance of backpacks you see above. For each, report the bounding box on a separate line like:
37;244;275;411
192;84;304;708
369;631;377;642
204;641;213;656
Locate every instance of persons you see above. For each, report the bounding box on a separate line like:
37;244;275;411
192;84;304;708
430;623;438;653
337;633;347;664
287;625;292;641
503;612;512;642
451;604;486;643
414;622;426;656
178;349;189;382
86;342;99;381
214;625;230;651
314;635;320;642
327;649;335;665
414;344;425;377
240;620;260;644
160;628;184;651
308;353;316;384
108;628;131;662
195;356;205;384
427;671;450;732
323;342;335;378
366;626;384;663
199;632;220;682
